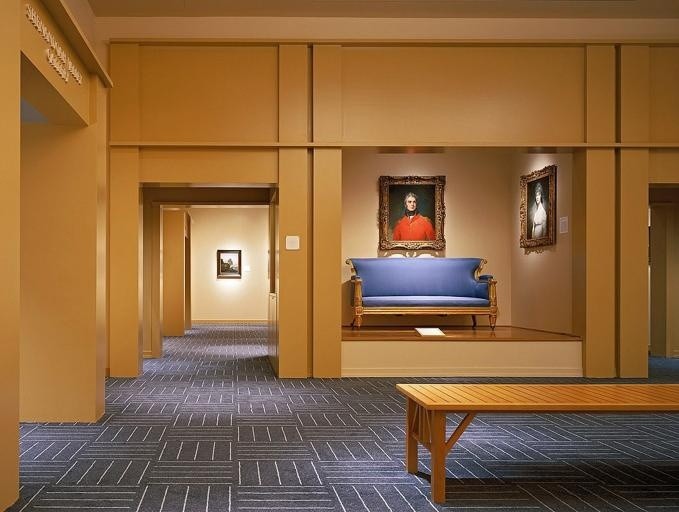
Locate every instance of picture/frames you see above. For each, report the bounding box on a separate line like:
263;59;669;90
379;175;446;250
519;164;557;248
217;250;241;279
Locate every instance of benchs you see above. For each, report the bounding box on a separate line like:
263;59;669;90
395;383;678;503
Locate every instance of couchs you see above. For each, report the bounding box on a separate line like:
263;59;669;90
346;258;498;331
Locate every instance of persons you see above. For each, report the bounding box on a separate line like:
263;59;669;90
530;182;548;238
394;191;435;241
